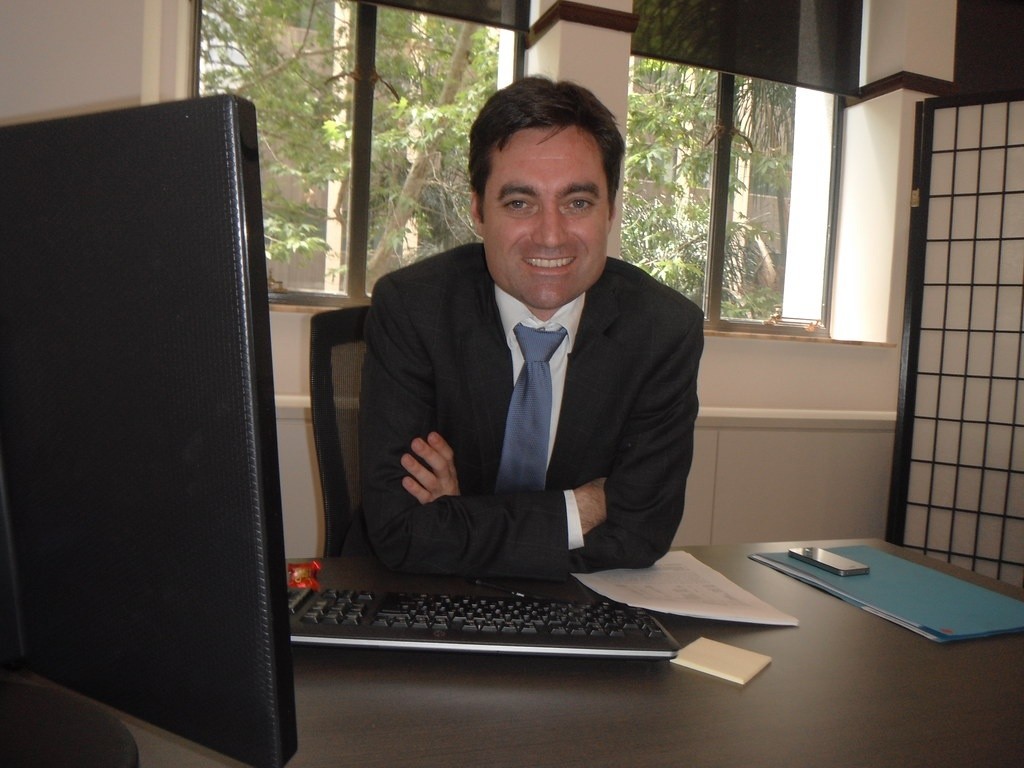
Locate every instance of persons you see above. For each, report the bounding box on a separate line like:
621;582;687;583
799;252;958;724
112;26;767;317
356;76;706;579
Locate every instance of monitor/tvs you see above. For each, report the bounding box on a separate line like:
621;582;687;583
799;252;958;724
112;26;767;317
0;93;299;768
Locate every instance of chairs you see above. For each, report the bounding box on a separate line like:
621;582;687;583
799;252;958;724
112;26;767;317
308;306;371;559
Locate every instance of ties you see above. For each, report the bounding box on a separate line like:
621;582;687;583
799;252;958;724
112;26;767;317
496;319;567;501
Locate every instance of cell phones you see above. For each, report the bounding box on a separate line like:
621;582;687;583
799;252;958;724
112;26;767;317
789;547;870;576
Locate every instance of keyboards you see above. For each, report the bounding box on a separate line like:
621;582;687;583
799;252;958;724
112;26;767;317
286;587;680;663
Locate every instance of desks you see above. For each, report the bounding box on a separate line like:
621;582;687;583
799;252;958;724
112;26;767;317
0;537;1024;768
